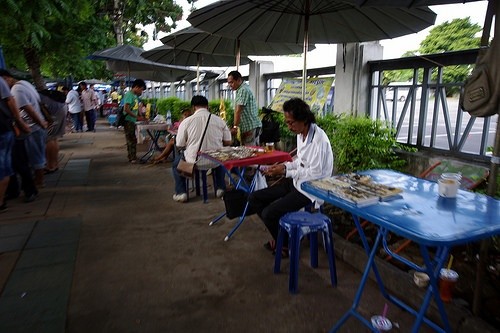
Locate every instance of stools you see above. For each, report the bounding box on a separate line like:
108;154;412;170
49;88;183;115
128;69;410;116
303;201;329;254
195;168;212;203
273;211;337;294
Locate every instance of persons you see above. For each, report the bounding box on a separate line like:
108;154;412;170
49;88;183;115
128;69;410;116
227;70;263;147
76;82;124;132
0;69;47;213
62;87;84;133
171;95;232;202
122;78;146;164
38;89;68;172
148;109;192;165
249;97;334;259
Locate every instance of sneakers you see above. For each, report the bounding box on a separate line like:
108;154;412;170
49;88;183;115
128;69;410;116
216;189;224;198
173;192;188;202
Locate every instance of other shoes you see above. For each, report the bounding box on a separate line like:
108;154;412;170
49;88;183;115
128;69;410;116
49;166;58;171
129;157;144;164
150;159;163;164
25;192;40;202
33;176;46;184
272;247;289;257
266;240;277;250
0;203;8;212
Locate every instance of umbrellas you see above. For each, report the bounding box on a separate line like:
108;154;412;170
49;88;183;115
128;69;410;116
79;72;138;86
140;44;254;95
0;46;9;72
160;0;317;72
186;0;437;101
87;43;215;91
10;70;32;80
45;80;78;90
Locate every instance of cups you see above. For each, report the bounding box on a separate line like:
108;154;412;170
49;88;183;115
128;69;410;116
266;143;274;152
371;315;392;333
439;268;459;303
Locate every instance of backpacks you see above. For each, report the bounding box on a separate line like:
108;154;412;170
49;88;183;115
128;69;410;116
38;89;66;104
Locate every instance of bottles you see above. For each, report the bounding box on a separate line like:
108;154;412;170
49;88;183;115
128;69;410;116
166;110;171;124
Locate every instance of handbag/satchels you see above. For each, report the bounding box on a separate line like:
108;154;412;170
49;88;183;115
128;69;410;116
224;188;256;219
259;113;281;143
176;159;195;179
39;103;54;127
0;98;16;135
255;170;268;191
115;109;126;127
459;0;500;117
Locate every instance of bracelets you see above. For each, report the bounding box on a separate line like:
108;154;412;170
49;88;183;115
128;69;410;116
233;126;238;130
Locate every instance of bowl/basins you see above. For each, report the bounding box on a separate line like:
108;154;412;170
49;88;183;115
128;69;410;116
438;179;462;198
413;271;430;288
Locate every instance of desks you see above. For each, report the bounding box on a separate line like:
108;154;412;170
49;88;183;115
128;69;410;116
136;123;172;164
300;169;500;333
197;145;293;243
